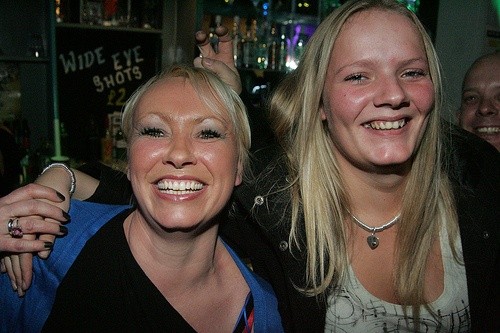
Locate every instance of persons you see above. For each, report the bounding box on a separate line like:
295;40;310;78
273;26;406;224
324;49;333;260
0;64;282;333
0;0;500;333
193;24;500;163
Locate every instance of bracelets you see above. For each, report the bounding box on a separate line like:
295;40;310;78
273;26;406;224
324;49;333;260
39;163;76;198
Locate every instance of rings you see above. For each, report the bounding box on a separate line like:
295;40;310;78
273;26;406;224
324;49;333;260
8;217;23;238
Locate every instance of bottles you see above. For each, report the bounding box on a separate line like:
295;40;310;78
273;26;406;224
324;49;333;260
208;15;307;70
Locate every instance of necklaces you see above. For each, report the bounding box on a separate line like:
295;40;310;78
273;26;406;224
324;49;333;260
342;204;402;249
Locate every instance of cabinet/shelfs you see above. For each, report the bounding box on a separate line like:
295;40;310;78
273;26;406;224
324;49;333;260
0;0;163;188
